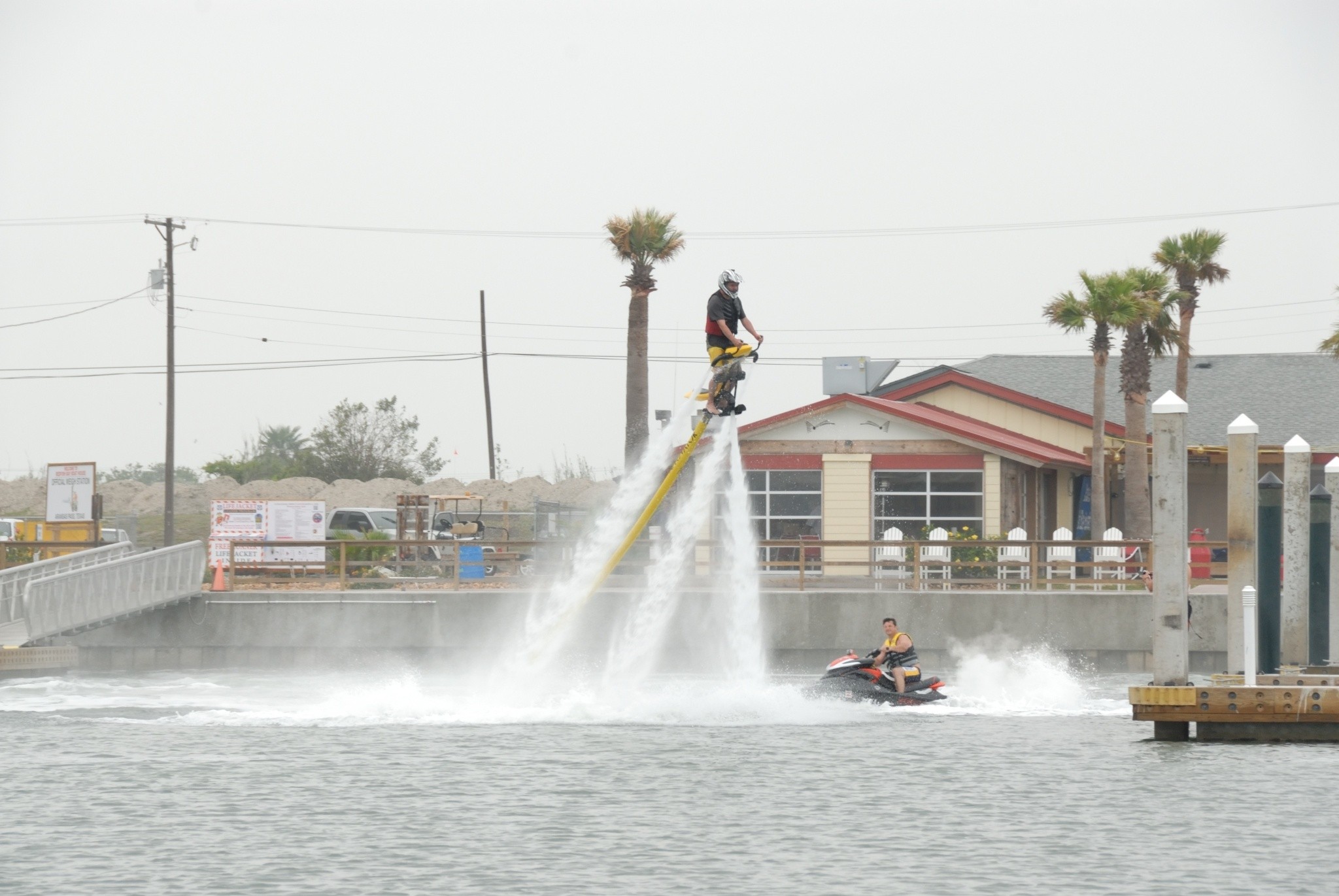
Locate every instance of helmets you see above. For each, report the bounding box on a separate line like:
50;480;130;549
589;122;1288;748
718;269;743;298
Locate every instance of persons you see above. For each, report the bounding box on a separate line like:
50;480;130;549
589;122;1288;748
873;618;922;693
705;269;764;416
1141;563;1192;629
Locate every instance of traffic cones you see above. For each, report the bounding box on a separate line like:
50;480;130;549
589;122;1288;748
209;558;230;592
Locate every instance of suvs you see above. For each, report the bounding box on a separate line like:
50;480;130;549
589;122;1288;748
324;506;498;577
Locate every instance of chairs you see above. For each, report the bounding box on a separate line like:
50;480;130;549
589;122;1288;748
761;533;821;570
919;527;952;590
1046;527;1076;591
997;527;1031;591
1093;527;1126;592
875;526;906;591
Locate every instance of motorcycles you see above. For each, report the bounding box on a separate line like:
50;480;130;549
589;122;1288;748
801;648;949;707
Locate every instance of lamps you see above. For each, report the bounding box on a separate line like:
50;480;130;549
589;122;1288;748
836;440;853;453
